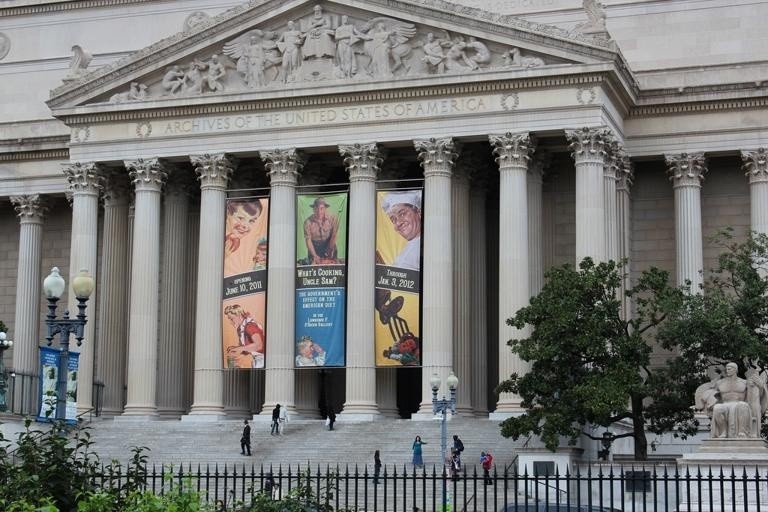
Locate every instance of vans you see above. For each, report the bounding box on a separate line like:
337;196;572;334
496;501;623;512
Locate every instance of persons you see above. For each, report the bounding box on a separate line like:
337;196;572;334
301;196;342;265
479;451;487;464
279;402;292;435
712;360;765;441
328;401;340;429
481;447;497;486
252;237;269;270
373;448;383;483
379;188;423;272
270;403;282;435
296;333;327;368
452;432;464;466
225;198;264;258
411;435;427;464
41;366;58;396
240;419;252;456
65;371;77;402
449;450;458;481
222;302;266;371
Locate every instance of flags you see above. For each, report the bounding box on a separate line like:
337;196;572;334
63;350;80;426
35;346;61;423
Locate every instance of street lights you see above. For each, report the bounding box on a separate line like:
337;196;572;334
429;370;460;477
40;263;96;473
598;427;615;466
0;330;16;413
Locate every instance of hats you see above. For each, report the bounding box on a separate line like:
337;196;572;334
309;198;330;208
381;190;421;213
